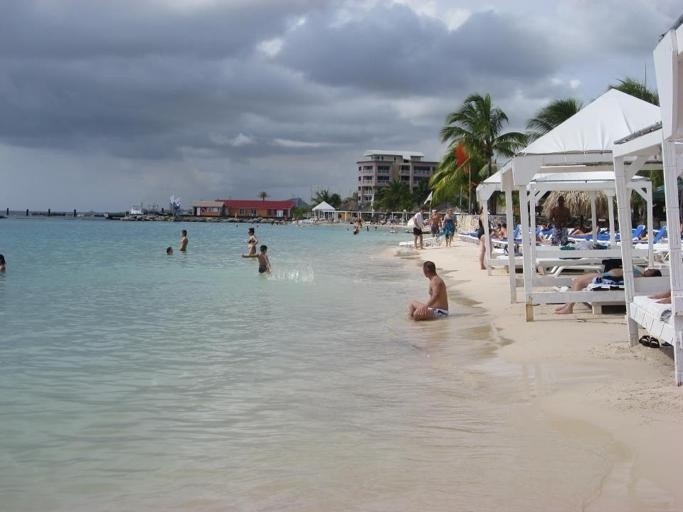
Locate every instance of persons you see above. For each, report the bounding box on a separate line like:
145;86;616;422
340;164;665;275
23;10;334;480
407;261;448;321
351;199;682;314
178;230;188;251
241;245;271;273
246;228;257;256
0;253;6;271
166;247;172;255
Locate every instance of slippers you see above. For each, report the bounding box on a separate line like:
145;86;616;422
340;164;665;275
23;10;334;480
639;335;659;347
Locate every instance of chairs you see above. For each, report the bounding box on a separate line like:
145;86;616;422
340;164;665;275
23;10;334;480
465;216;682;383
